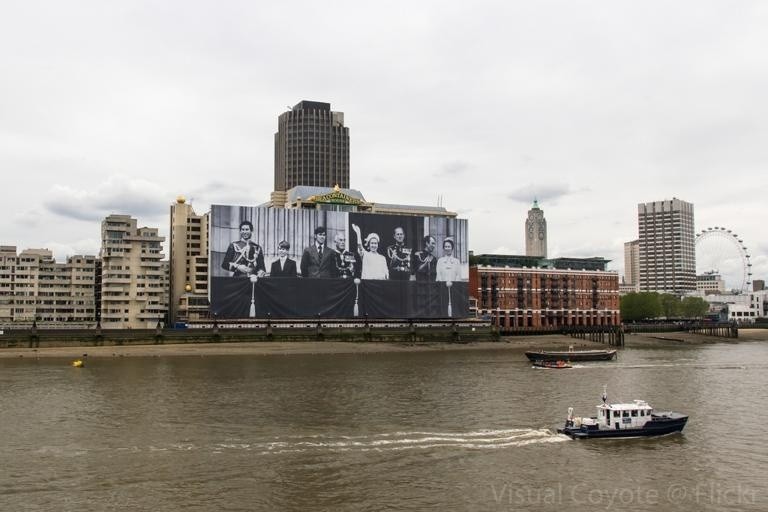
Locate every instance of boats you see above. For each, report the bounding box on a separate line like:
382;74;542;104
72;360;84;367
557;380;690;442
525;346;618;362
531;362;572;369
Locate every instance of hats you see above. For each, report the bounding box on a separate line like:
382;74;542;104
364;233;380;247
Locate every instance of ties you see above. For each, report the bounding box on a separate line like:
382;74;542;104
318;246;323;257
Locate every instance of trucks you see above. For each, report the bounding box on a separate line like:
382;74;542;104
175;321;188;328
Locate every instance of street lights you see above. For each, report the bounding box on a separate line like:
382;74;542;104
95;312;102;329
248;273;257;318
364;312;369;327
32;312;38;327
447;281;454;317
318;312;322;326
354;278;361;318
214;312;217;326
268;312;271;325
156;312;163;328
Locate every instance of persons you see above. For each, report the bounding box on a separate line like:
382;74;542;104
220;220;464;281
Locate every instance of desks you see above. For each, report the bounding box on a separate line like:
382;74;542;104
211;276;468;319
